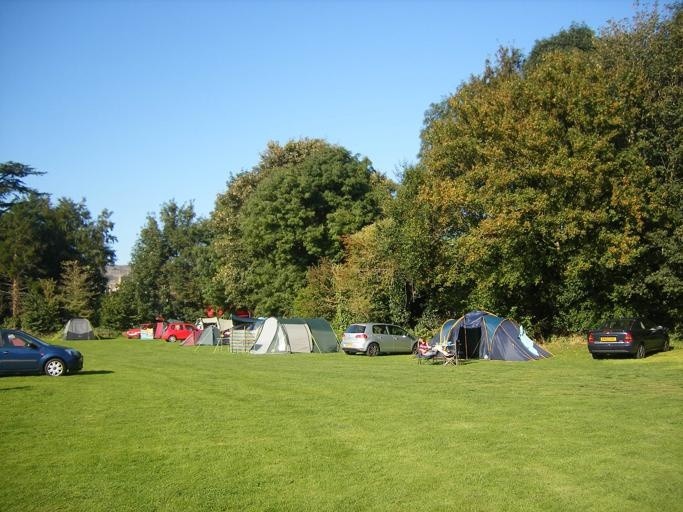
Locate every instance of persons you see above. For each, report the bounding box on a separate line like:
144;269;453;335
419;338;454;358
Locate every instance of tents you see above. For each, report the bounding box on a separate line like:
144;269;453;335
179;325;221;346
430;311;553;361
63;319;97;340
248;317;341;354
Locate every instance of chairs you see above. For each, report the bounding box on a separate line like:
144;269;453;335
418;350;439;365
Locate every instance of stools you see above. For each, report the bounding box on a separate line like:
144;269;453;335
439;350;457;367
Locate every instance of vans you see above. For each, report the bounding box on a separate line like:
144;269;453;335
338;321;421;357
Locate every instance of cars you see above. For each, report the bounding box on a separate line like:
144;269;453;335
0;328;84;375
585;315;670;358
161;322;202;342
126;323;156;340
223;323;246;340
